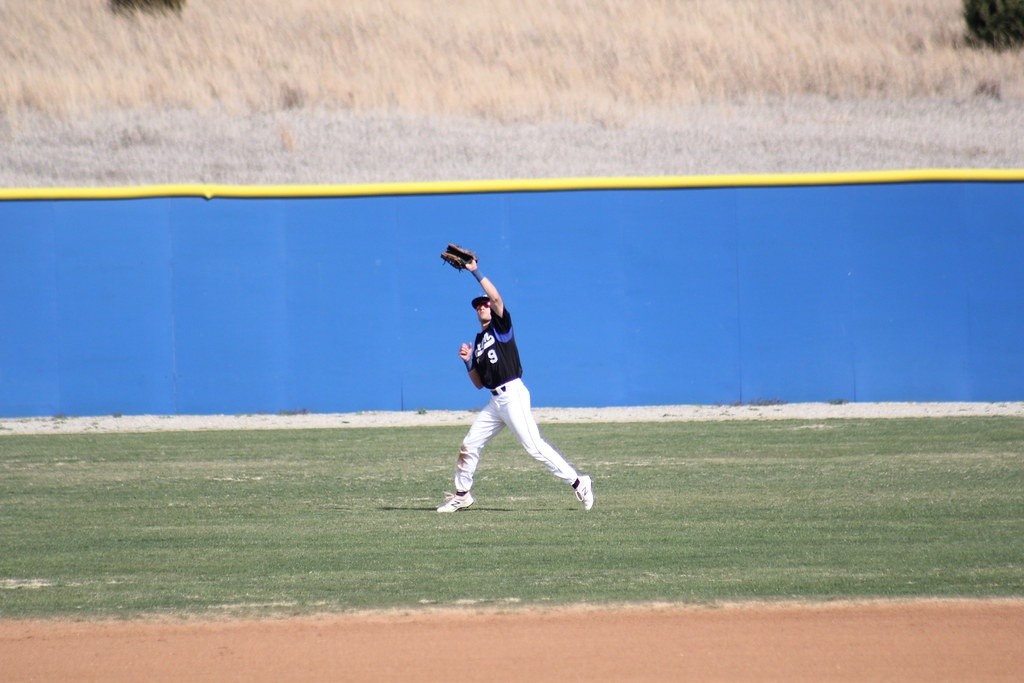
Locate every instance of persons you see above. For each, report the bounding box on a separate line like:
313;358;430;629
436;244;594;513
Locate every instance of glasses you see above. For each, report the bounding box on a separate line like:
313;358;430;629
474;299;488;306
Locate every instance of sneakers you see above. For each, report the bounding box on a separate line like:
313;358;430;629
575;475;594;511
436;492;474;513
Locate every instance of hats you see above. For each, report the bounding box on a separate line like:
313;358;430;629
472;294;490;309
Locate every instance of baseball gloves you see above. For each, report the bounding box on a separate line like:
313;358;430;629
440;243;478;271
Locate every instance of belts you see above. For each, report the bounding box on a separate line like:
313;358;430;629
491;386;505;395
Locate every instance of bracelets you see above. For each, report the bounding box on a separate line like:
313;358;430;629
464;356;475;372
470;268;485;282
